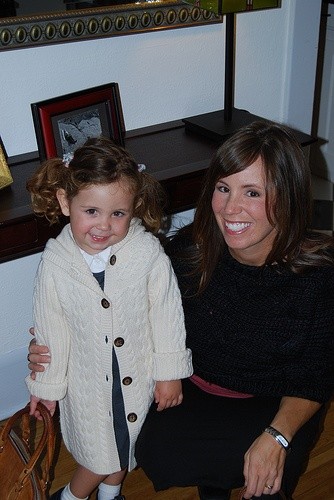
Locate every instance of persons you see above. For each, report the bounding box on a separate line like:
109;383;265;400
26;136;194;500
27;121;334;500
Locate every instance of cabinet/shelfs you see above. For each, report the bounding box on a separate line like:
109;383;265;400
0;0;318;265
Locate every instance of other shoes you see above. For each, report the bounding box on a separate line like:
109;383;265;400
51;487;64;500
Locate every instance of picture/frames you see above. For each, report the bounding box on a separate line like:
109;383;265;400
31;83;127;167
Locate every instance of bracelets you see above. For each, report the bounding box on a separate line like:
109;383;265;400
264;425;292;452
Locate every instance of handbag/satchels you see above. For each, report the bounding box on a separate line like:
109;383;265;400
0;401;55;500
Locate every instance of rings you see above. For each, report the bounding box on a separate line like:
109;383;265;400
27;353;30;362
265;483;273;489
